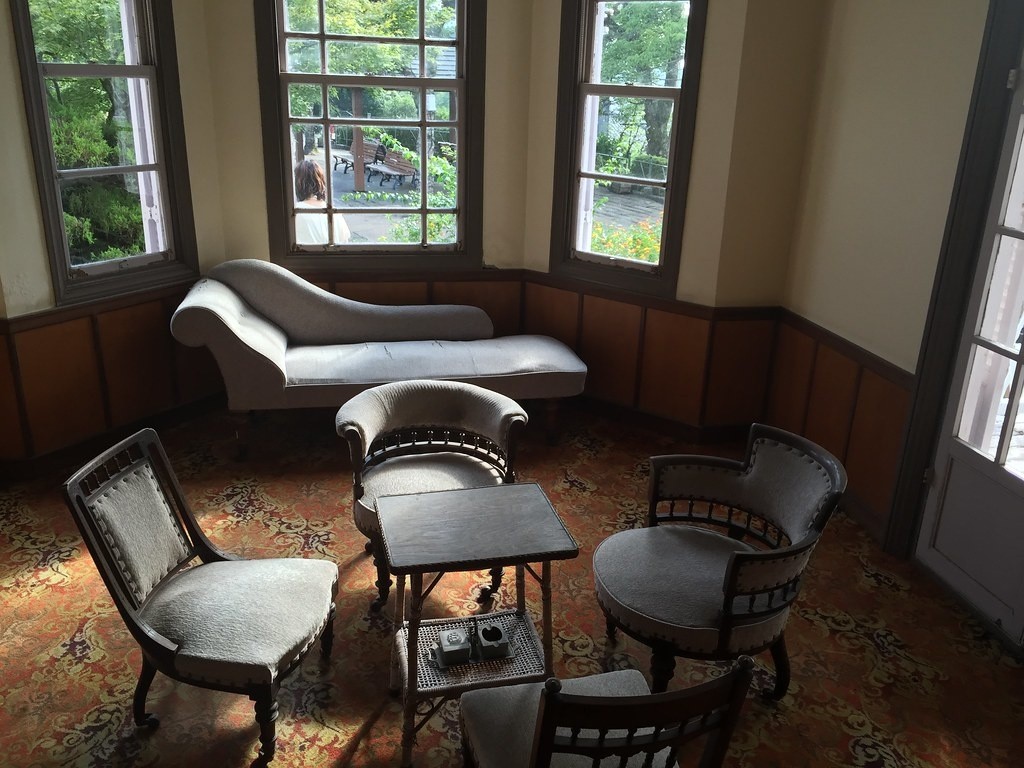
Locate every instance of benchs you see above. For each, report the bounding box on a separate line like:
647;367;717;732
333;139;419;190
168;259;589;455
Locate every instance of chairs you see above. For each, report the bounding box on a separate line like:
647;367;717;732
333;379;531;615
591;424;848;704
64;428;338;768
458;654;756;768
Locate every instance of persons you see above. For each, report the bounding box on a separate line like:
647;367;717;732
292;158;351;245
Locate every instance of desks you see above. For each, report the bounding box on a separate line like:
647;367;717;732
372;480;580;767
